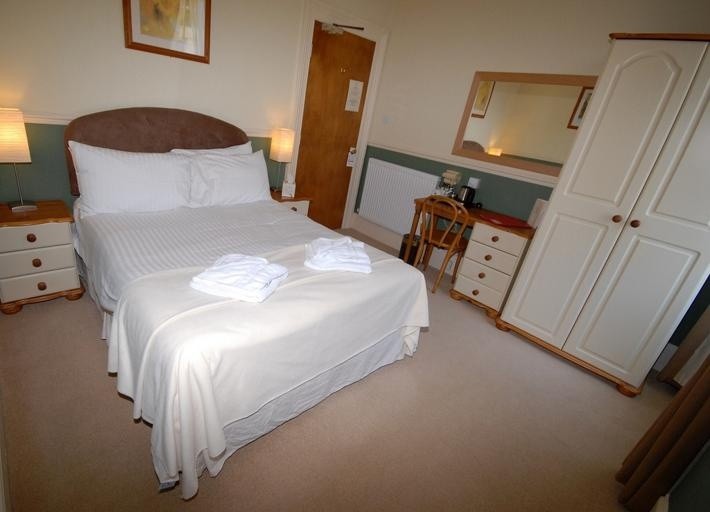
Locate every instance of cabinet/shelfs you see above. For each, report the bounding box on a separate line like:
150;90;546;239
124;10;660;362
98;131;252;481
492;31;710;398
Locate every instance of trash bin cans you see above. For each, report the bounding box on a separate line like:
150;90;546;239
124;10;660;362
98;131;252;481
399;234;421;265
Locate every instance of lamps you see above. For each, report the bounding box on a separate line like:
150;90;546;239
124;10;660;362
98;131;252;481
269;127;296;193
0;107;39;213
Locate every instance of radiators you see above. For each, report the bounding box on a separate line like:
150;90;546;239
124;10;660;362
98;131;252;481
358;157;441;236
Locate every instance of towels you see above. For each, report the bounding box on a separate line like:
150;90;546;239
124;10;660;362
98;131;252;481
188;253;289;303
303;235;372;275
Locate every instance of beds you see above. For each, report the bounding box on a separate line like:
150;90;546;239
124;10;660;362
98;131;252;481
63;107;430;502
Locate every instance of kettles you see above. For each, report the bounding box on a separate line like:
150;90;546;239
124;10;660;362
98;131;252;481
458;185;476;207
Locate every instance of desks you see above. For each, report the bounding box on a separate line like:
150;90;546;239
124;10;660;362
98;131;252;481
403;196;537;319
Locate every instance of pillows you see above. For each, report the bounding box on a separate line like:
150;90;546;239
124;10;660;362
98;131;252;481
170;139;253;157
187;148;272;212
66;140;189;220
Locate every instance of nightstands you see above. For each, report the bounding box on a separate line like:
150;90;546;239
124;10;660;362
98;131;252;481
271;189;313;217
0;199;85;315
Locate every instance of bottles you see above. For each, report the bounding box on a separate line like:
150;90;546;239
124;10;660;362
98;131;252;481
438;181;455;199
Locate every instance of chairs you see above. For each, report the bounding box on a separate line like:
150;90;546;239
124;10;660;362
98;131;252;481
412;194;469;294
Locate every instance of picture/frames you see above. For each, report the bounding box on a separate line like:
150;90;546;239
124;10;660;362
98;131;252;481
471;80;495;118
122;0;211;64
567;85;595;129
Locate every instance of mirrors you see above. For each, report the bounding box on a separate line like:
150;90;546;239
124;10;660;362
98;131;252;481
450;71;599;177
